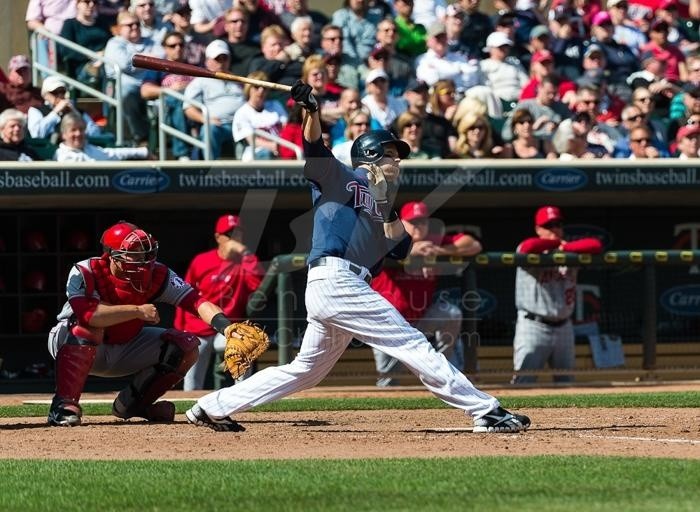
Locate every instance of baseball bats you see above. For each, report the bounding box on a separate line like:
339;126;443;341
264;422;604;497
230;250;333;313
132;54;293;91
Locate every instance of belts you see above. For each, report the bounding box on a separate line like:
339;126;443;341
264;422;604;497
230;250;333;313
309;257;371;283
526;314;569;328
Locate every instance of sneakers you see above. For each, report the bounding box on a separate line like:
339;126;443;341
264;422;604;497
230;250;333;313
112;394;171;422
47;400;81;426
473;408;531;434
185;402;246;431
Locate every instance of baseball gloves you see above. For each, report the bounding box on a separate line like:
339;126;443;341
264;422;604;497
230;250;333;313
225;324;268;379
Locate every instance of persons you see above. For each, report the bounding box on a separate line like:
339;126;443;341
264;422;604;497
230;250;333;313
1;2;699;161
367;199;483;388
181;80;532;432
510;203;605;384
171;212;265;390
43;220;244;427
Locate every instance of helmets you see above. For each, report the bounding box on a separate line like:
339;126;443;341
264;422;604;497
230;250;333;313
100;221;159;296
350;130;411;171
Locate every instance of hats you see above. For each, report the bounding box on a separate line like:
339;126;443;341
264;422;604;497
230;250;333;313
486;1;628;87
400;201;429;221
174;0;191;13
569;111;592;121
639;0;682;61
40;74;68;97
404;78;427;92
7;54;31;73
426;21;447;38
675;125;700;141
205;40;230;60
367;67;389;84
215;216;244;235
535;206;564;226
